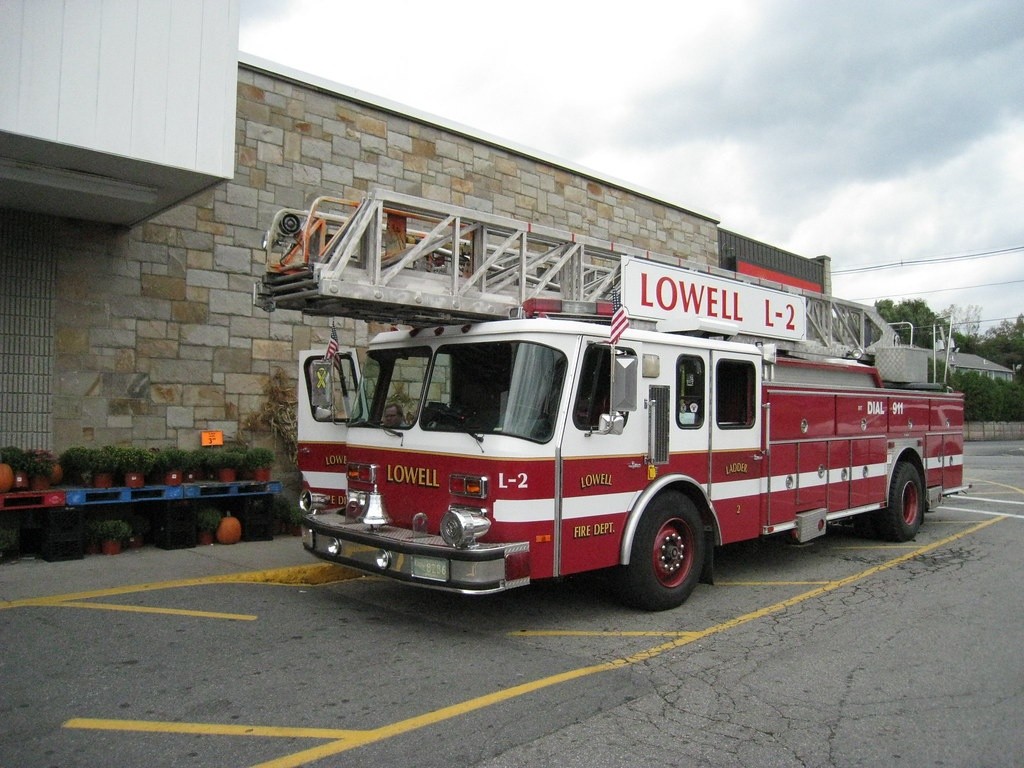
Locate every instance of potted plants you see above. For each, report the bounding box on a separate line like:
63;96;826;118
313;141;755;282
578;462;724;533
0;439;311;562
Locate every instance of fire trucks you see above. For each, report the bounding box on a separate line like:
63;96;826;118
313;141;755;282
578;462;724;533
252;188;973;610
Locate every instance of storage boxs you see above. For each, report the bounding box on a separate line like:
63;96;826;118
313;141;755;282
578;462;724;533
874;345;929;384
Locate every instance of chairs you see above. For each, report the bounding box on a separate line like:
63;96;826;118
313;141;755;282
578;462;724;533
451;361;499;432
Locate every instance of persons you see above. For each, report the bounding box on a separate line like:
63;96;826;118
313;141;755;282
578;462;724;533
380;402;406;429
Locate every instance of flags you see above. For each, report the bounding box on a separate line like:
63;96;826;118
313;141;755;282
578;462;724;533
324;324;339;360
611;288;629;345
